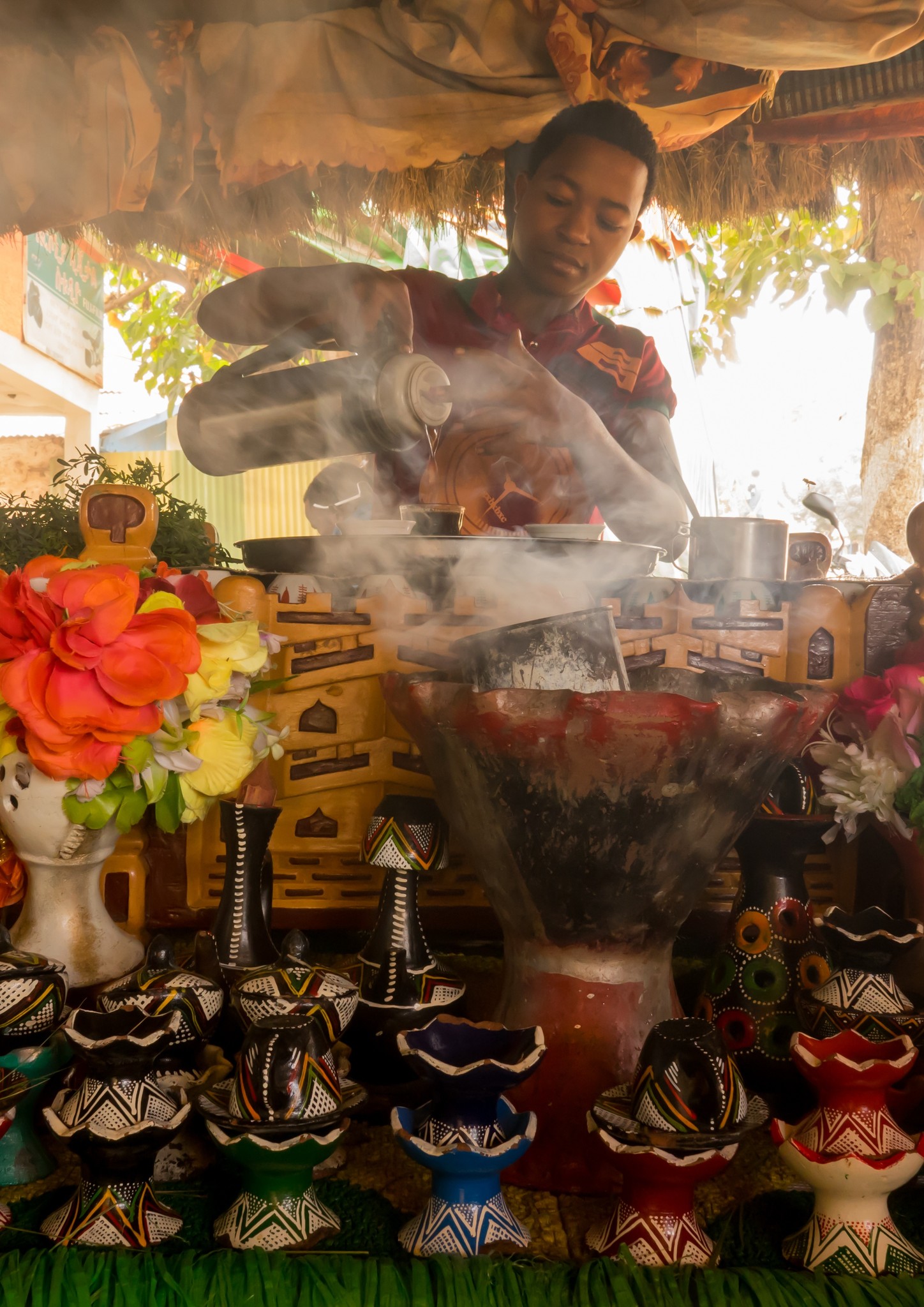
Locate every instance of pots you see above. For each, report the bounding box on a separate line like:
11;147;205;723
449;605;631;693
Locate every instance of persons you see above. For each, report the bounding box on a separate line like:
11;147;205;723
195;98;694;572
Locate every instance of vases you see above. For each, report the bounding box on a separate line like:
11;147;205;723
0;749;143;985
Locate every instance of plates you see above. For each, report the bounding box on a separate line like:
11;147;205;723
196;1077;372;1135
591;1084;773;1151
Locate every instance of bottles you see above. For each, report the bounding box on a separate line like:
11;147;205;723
686;814;837;1122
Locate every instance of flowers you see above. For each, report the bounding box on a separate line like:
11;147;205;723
0;555;293;829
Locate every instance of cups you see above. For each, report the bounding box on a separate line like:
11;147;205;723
227;1014;342;1120
629;1016;748;1134
669;517;788;581
399;503;466;535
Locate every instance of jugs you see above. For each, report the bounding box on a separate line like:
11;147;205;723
176;324;453;477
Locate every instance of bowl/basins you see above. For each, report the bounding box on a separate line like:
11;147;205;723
523;524;606;541
335;519;416;535
